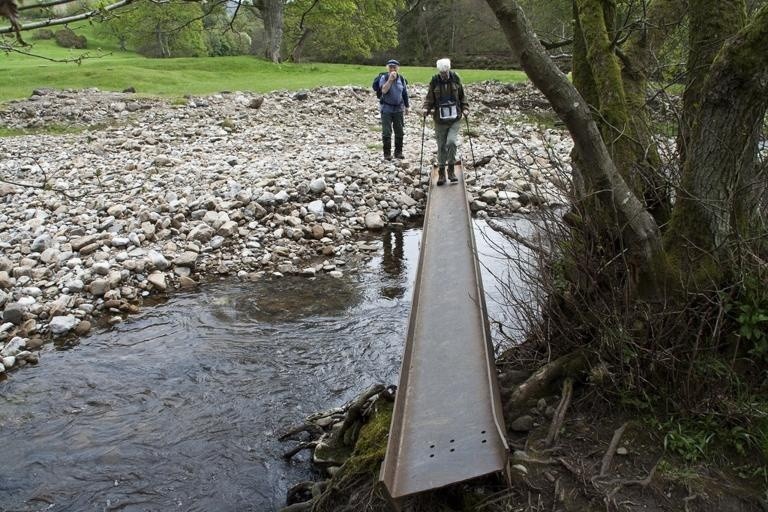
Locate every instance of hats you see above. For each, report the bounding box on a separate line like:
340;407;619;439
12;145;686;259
386;60;400;66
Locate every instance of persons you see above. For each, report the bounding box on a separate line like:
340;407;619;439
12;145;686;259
379;60;409;160
423;57;469;186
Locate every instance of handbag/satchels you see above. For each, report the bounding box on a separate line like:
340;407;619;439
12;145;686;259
433;100;464;124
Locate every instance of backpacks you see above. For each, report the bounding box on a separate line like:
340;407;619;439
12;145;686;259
372;72;409;101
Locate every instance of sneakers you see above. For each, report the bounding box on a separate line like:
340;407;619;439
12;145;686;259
392;150;405;158
383;150;392;161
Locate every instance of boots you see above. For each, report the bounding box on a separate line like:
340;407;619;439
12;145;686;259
436;169;447;185
447;168;458;184
